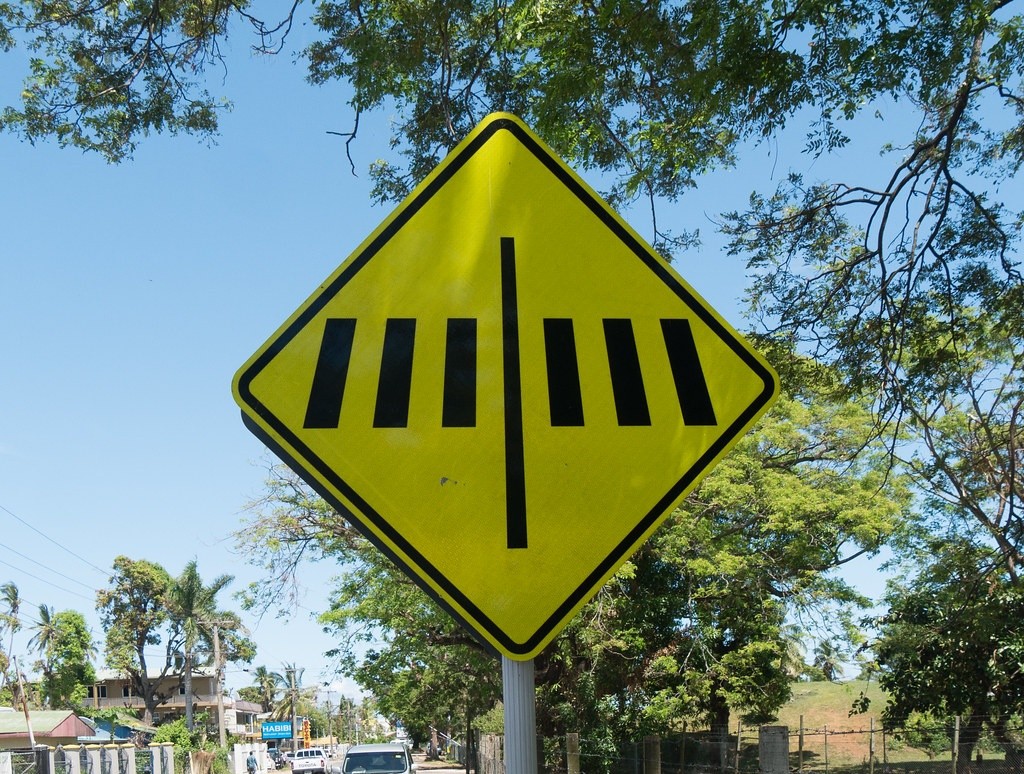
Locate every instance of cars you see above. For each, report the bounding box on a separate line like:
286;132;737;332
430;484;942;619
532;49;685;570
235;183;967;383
426;743;442;756
267;748;295;770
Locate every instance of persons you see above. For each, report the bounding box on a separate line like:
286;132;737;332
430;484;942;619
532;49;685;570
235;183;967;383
247;750;258;774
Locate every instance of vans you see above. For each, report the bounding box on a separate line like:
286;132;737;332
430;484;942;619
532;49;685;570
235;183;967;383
332;744;419;774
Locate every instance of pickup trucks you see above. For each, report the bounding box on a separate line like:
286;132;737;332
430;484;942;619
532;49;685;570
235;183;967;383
290;748;332;774
